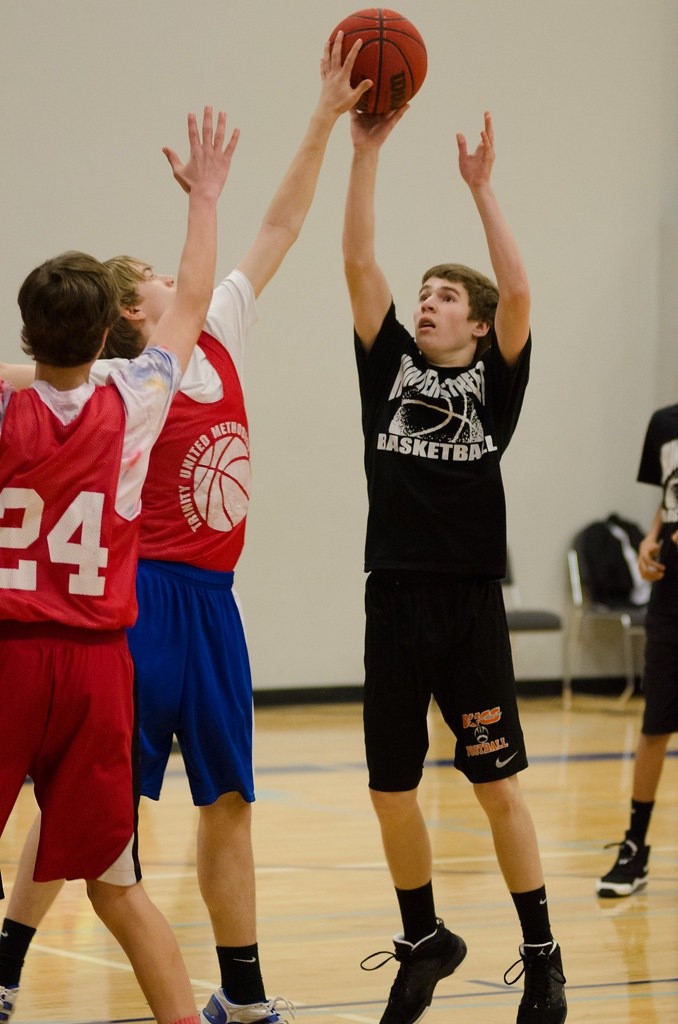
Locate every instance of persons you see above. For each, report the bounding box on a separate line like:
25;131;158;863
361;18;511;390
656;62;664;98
341;103;568;1024
0;104;244;1024
0;28;375;1024
596;404;678;899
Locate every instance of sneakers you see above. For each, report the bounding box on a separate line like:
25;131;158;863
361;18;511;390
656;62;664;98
0;983;20;1024
503;939;568;1024
595;830;651;897
360;917;467;1024
200;987;297;1024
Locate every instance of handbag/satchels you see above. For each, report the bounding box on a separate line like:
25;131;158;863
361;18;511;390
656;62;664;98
581;513;654;609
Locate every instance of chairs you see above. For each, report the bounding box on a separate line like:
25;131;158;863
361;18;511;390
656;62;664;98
561;517;648;714
497;550;569;710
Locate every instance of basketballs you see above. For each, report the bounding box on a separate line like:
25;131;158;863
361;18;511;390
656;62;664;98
326;8;428;114
